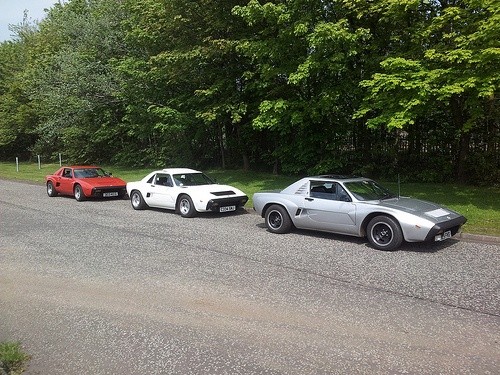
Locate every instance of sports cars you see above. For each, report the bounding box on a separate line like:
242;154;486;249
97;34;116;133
126;166;249;218
251;175;468;252
45;166;127;202
